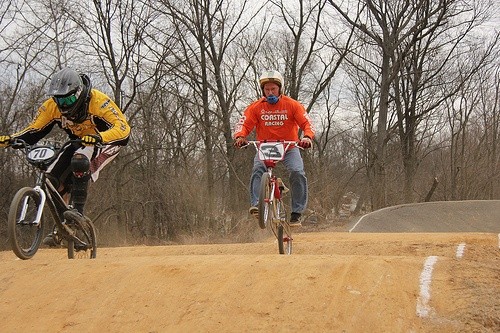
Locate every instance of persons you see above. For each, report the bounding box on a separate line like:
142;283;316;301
0;67;131;246
233;70;315;226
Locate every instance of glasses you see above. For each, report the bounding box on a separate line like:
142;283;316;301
52;91;77;107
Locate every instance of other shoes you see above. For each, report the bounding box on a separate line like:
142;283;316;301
249;207;259;220
64;209;86;230
289;212;302;227
42;232;61;247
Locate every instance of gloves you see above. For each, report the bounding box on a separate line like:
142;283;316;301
233;136;248;148
0;135;10;148
298;137;312;148
81;134;102;146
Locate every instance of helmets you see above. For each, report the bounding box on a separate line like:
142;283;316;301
259;70;284;96
46;69;84;107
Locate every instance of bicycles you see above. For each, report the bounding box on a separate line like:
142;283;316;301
235;138;310;254
0;135;100;261
